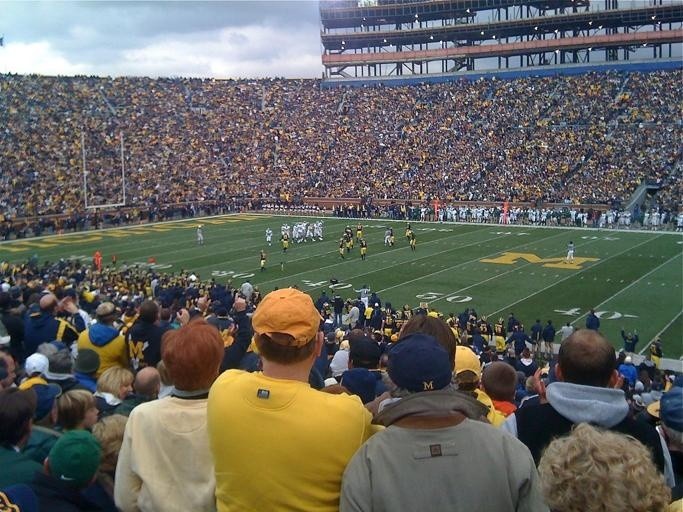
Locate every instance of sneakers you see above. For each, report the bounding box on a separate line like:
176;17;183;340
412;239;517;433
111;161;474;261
341;367;376;404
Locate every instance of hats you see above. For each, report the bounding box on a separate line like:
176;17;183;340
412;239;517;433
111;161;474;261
17;377;64;421
23;353;48;377
349;336;385;368
384;333;457;391
47;430;103;491
73;348;100;374
624;357;632;363
249;286;324;351
659;386;683;433
1;336;17;349
451;346;482;376
